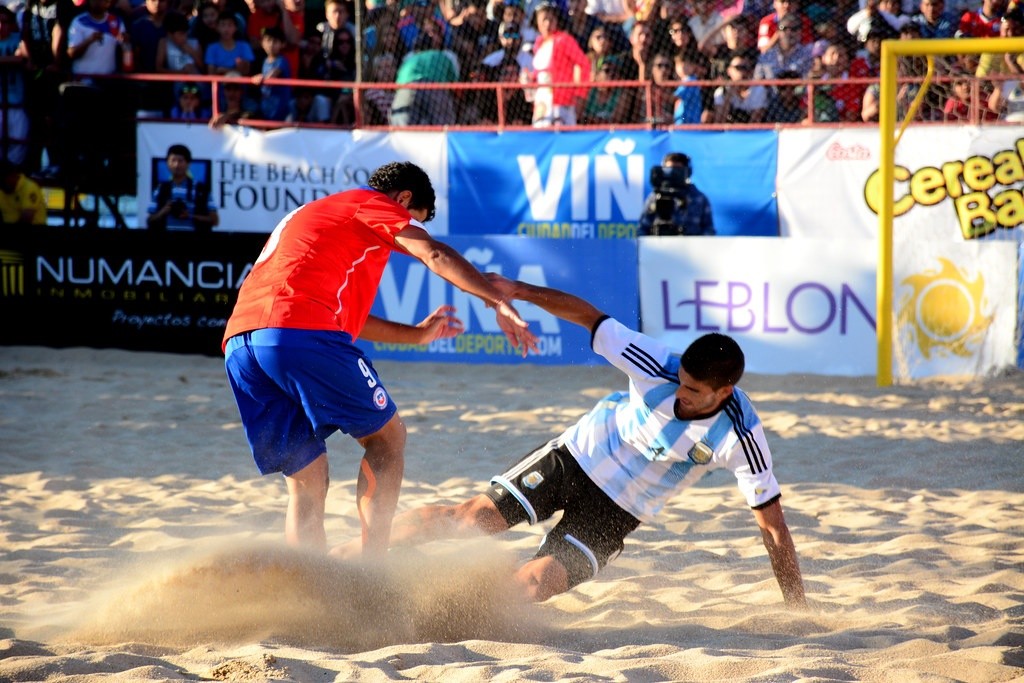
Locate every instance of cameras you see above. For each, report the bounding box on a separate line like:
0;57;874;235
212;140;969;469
169;198;188;214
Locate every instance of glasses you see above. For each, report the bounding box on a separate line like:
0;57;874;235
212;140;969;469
181;87;196;93
604;69;619;73
674;29;692;32
504;32;518;39
779;24;802;31
653;63;670;69
498;69;519;77
728;63;752;73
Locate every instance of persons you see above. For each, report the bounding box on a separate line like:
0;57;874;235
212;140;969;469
146;143;221;232
328;270;813;616
0;141;45;227
635;151;717;235
0;0;1023;129
221;160;543;578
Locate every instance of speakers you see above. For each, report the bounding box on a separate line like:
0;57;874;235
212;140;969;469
59;83;138;197
